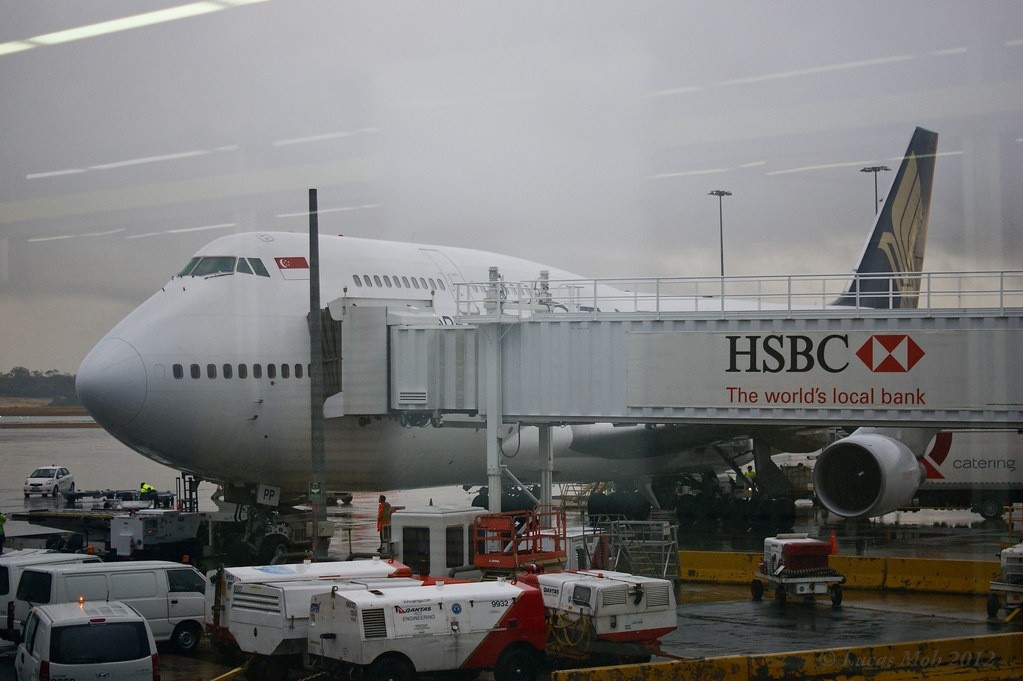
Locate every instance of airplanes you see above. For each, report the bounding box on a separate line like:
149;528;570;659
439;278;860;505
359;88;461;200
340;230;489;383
73;124;941;533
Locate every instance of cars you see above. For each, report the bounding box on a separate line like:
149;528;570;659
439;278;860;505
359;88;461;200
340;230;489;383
23;465;76;497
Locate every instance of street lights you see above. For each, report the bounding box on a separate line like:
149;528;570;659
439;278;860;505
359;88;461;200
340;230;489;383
860;165;892;213
709;188;728;312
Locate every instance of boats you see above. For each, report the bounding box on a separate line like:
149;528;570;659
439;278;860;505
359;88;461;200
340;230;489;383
118;498;152;510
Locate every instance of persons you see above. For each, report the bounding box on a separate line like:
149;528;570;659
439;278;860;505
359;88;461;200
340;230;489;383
743;466;755;479
377;495;391;551
0;513;6;554
140;483;160;508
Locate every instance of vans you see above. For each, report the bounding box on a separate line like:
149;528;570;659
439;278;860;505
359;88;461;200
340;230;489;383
11;598;162;681
0;553;107;633
11;559;209;654
